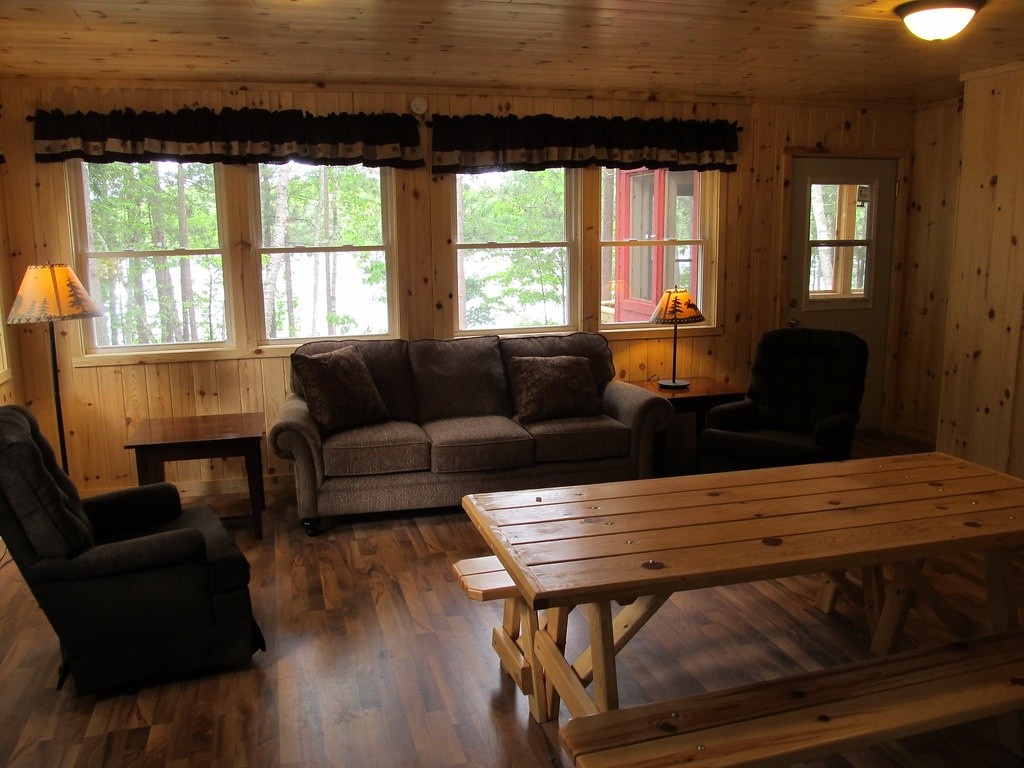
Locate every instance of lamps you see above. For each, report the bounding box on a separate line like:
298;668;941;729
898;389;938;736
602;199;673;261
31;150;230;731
3;262;103;475
647;285;706;389
892;0;986;42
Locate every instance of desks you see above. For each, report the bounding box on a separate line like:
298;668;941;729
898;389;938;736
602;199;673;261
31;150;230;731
124;411;265;543
630;376;746;483
462;453;1024;724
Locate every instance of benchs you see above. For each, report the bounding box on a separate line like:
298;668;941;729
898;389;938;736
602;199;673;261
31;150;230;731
452;552;518;679
558;623;1024;768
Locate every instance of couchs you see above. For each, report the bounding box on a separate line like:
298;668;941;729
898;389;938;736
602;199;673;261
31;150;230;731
1;404;266;696
267;329;677;536
693;327;868;476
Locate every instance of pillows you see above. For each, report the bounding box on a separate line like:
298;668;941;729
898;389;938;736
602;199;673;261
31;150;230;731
509;356;608;422
290;345;391;436
